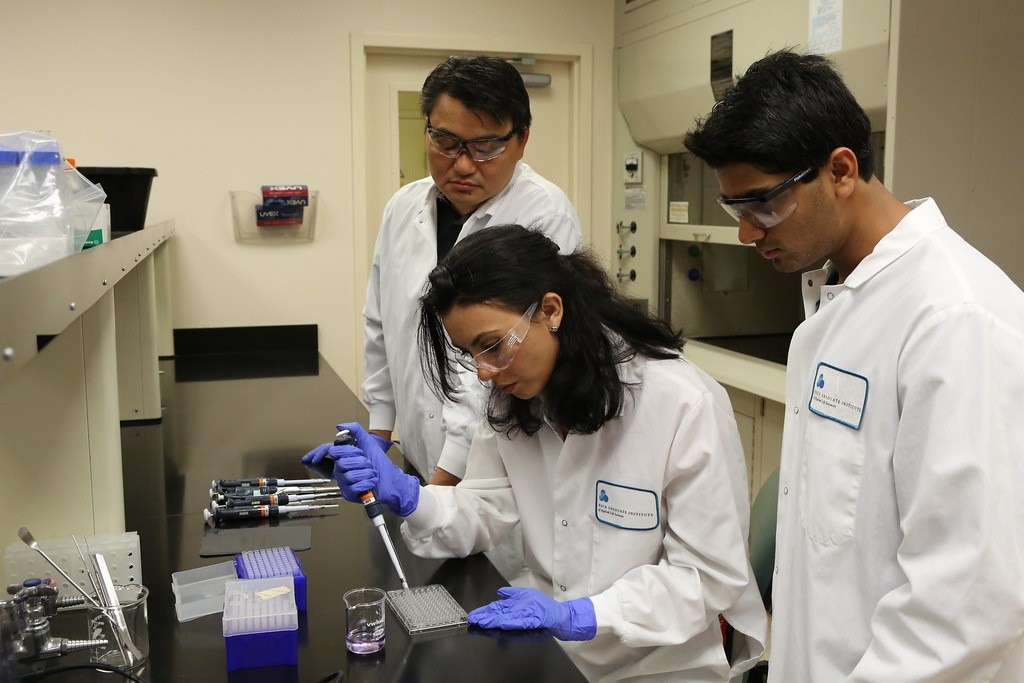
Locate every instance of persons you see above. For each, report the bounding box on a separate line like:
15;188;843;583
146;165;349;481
675;43;1024;683
326;221;773;682
301;54;581;494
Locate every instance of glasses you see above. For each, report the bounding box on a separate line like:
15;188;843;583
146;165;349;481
423;116;524;161
717;158;830;224
456;296;541;374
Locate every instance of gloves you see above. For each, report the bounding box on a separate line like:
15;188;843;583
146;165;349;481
302;433;393;468
330;423;419;518
465;587;597;641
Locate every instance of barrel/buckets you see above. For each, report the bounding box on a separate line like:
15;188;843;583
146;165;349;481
0;149;78;275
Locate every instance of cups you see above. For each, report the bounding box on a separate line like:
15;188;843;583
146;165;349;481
84;584;151;673
343;588;387;653
